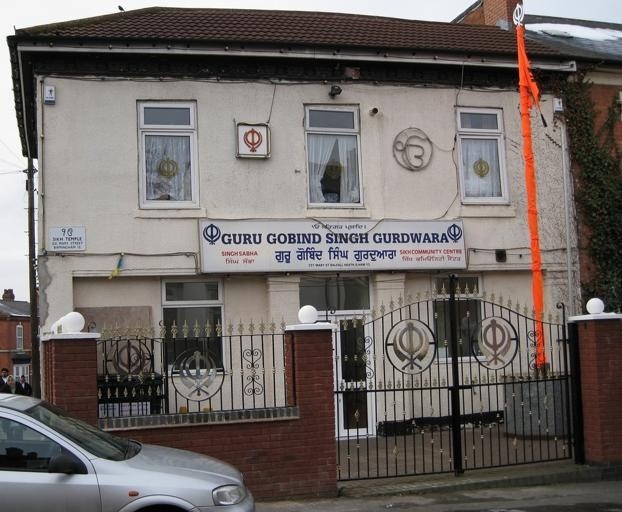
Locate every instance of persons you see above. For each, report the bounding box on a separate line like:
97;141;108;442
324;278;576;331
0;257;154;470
19;375;32;395
0;375;25;395
0;368;11;387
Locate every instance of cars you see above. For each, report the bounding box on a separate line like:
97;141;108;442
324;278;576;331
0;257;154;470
0;391;254;512
